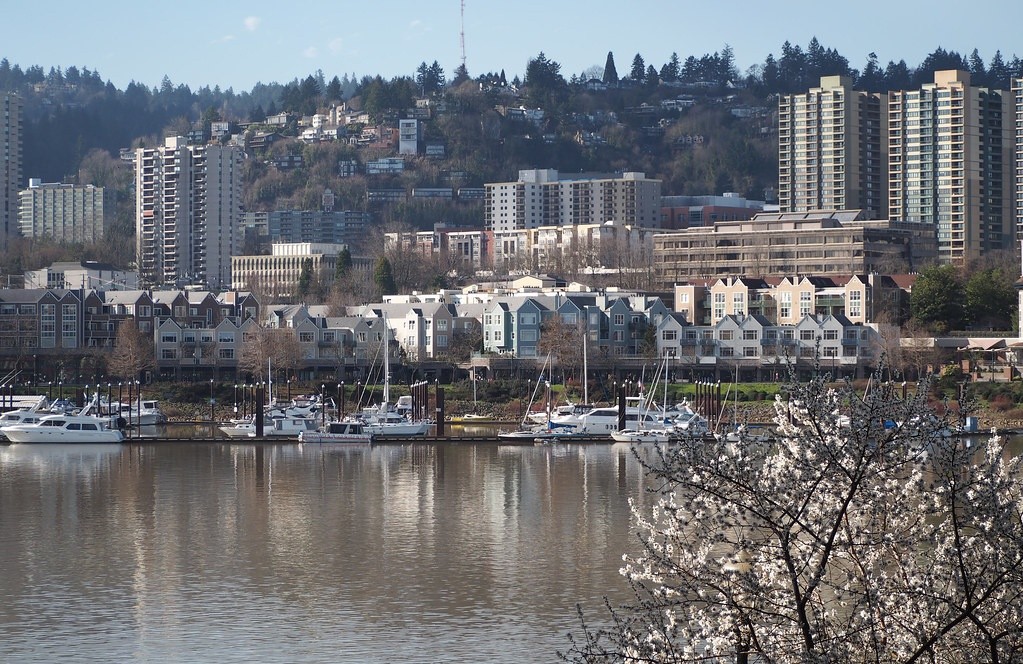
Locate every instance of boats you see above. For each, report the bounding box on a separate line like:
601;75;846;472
881;412;963;438
218;393;433;443
0;396;160;443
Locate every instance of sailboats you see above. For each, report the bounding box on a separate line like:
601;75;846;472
497;333;770;443
